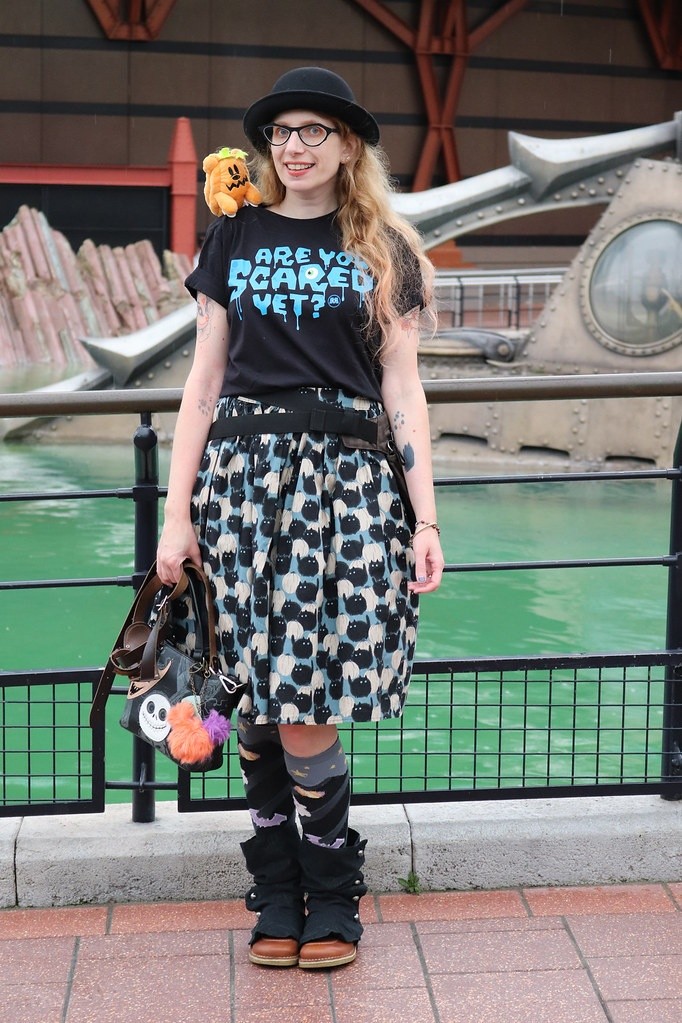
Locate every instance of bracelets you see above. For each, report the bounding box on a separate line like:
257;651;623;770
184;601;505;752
408;523;437;546
415;521;440;536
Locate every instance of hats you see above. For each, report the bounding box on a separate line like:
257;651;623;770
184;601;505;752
243;65;382;160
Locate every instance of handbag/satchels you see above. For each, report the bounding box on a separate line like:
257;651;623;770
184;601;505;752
88;556;247;773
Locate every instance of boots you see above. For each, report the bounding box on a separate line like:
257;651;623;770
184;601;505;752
296;825;369;969
238;824;306;968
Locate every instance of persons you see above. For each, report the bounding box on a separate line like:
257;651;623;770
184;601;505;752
156;65;446;969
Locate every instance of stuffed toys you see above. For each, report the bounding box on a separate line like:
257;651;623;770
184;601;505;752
203;147;262;217
162;695;233;766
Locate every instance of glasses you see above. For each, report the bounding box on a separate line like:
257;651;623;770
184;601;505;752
255;122;341;147
